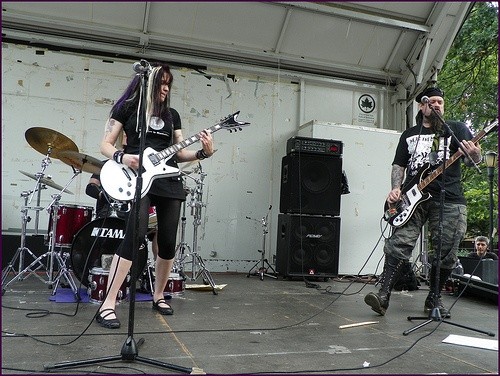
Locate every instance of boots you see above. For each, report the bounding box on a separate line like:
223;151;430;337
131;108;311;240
424;268;453;317
363;254;406;317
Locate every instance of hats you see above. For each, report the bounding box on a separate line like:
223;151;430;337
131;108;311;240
416;88;445;103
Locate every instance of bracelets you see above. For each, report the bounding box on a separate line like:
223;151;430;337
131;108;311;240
113;151;123;164
469;155;482;165
195;149;210;160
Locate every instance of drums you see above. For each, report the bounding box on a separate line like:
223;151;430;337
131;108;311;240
151;271;185;298
148;206;158;228
46;203;94;248
87;267;130;305
71;216;149;290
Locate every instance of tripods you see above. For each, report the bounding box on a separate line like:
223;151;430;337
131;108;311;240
247;217;278;280
45;75;206;374
174;171;218;294
2;155;82;302
402;107;495;336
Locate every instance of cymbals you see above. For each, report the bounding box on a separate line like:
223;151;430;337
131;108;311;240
19;170;73;194
57;150;104;175
26;127;79;159
180;149;218;170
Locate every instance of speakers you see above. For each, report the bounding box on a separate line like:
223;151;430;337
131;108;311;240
275;153;342;216
277;214;341;281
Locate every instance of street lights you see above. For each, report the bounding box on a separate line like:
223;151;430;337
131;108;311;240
484;150;498;253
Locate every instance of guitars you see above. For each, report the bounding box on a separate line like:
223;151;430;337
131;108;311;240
384;116;499;228
99;111;251;203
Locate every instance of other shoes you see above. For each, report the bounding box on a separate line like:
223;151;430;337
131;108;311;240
152;298;173;315
96;308;121;329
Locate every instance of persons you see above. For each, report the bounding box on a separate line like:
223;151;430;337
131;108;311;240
365;87;483;318
86;130;157;261
85;59;215;328
467;236;498;260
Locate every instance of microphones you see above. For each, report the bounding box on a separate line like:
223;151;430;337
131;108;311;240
133;62;149;73
421;96;433;110
